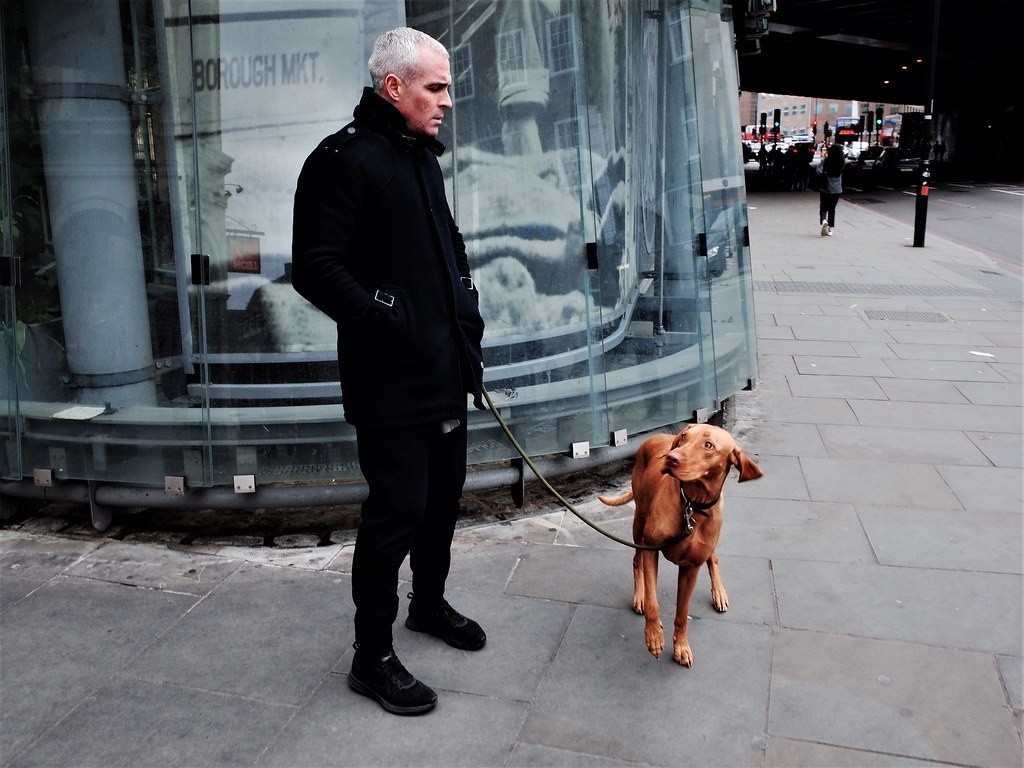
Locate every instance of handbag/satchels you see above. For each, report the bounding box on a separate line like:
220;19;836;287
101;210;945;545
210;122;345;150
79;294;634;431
817;173;829;190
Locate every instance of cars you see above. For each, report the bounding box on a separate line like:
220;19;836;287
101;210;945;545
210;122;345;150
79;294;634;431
685;203;747;277
739;124;938;196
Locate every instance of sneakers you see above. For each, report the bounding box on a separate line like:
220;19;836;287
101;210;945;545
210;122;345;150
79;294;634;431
347;642;438;715
828;228;835;236
405;592;486;651
821;220;828;236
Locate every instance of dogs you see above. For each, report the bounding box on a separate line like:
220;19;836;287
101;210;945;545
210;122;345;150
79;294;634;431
596;422;767;671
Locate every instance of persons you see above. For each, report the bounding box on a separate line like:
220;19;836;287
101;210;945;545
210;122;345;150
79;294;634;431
755;141;816;193
291;27;488;717
816;144;845;237
